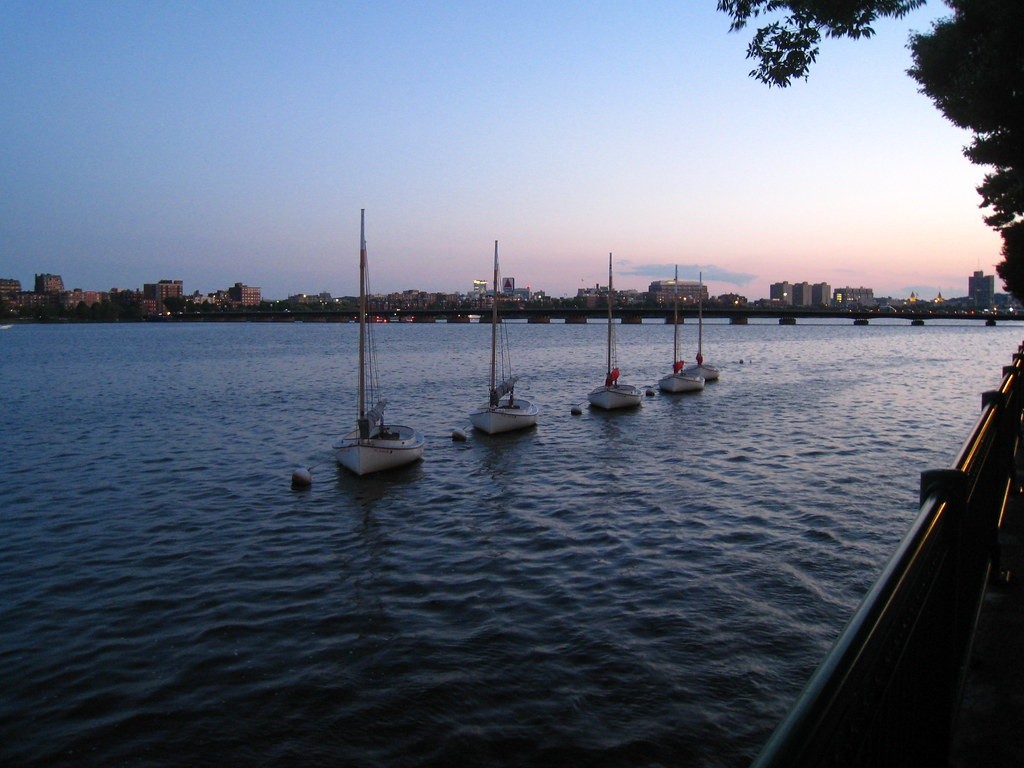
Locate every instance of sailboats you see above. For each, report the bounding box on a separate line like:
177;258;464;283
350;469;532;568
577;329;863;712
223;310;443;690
587;253;643;412
657;265;706;396
467;240;541;437
683;272;720;381
331;208;429;478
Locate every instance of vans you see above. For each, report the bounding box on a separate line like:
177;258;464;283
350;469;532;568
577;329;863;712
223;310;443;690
879;306;896;313
